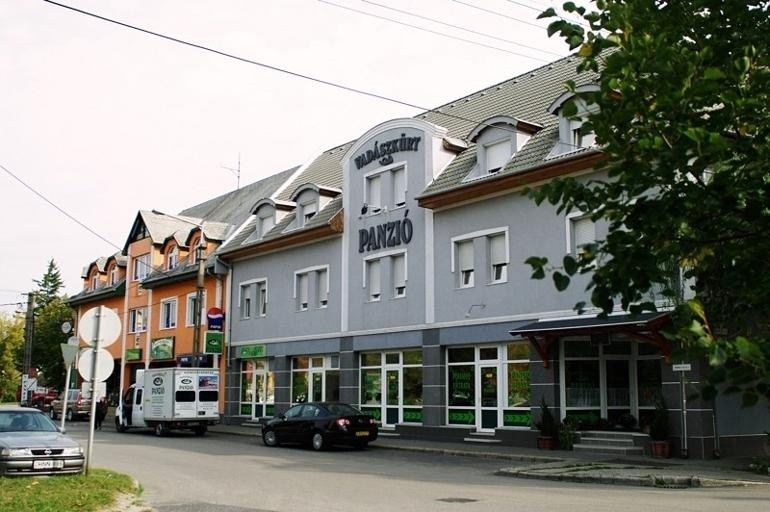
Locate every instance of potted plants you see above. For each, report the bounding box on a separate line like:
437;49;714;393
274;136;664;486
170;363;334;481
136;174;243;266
533;394;560;450
646;401;672;458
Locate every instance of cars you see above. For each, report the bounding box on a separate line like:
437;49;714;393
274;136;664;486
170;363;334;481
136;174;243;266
1;404;85;476
262;400;378;451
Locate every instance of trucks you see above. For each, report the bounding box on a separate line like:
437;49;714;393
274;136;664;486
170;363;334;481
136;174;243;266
114;367;220;436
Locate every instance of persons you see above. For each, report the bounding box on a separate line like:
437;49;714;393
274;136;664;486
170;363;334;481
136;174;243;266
95;397;108;430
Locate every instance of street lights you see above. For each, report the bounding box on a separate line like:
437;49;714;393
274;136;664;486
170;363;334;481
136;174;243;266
151;209;207;367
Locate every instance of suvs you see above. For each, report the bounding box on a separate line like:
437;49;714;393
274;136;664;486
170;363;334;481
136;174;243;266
49;389;92;421
